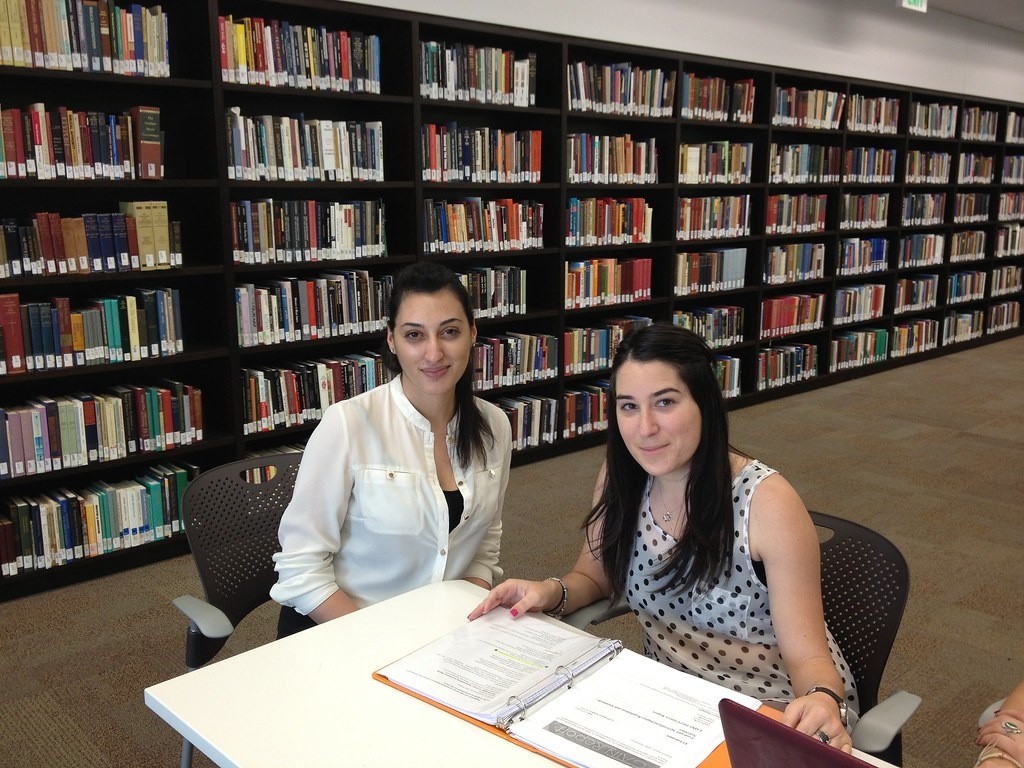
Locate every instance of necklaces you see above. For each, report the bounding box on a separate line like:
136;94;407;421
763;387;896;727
655;479;687;522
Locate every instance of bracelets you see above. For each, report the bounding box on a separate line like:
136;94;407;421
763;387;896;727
543;576;568;618
805;686;848;724
974;742;1024;768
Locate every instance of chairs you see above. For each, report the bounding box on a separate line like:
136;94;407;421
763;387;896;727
558;510;923;768
181;451;302;768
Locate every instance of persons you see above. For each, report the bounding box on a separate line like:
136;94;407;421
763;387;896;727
467;325;852;754
269;262;512;640
974;676;1024;768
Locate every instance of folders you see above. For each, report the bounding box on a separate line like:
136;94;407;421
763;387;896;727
370;606;786;768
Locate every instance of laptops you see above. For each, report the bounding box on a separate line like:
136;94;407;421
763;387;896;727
717;699;878;768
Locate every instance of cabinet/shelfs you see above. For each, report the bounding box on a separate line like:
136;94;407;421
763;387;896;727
0;0;1023;600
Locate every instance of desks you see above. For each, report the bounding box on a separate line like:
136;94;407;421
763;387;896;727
144;581;783;768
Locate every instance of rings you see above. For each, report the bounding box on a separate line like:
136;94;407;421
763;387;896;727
1003;722;1021;734
815;730;829;744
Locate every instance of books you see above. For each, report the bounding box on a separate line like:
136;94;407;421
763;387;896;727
376;604;762;768
0;0;1024;575
